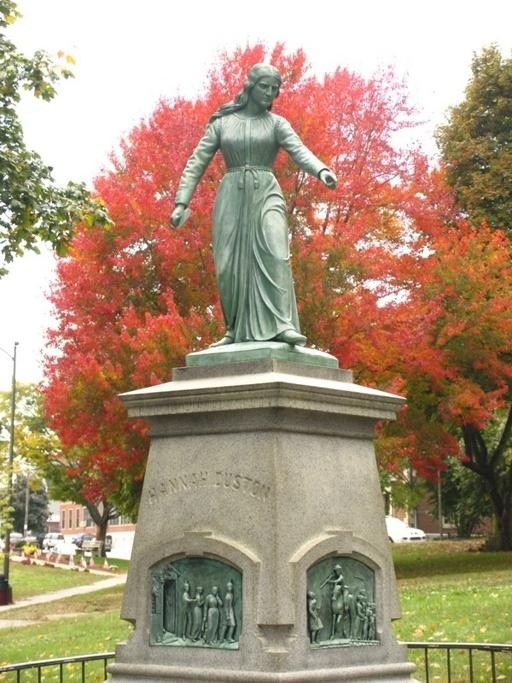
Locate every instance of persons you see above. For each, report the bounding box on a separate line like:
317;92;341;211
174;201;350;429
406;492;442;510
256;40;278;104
326;563;375;642
182;578;235;645
307;590;323;644
168;63;337;349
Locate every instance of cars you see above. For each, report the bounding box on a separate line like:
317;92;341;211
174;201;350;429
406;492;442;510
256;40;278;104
385;514;428;543
3;529;111;553
